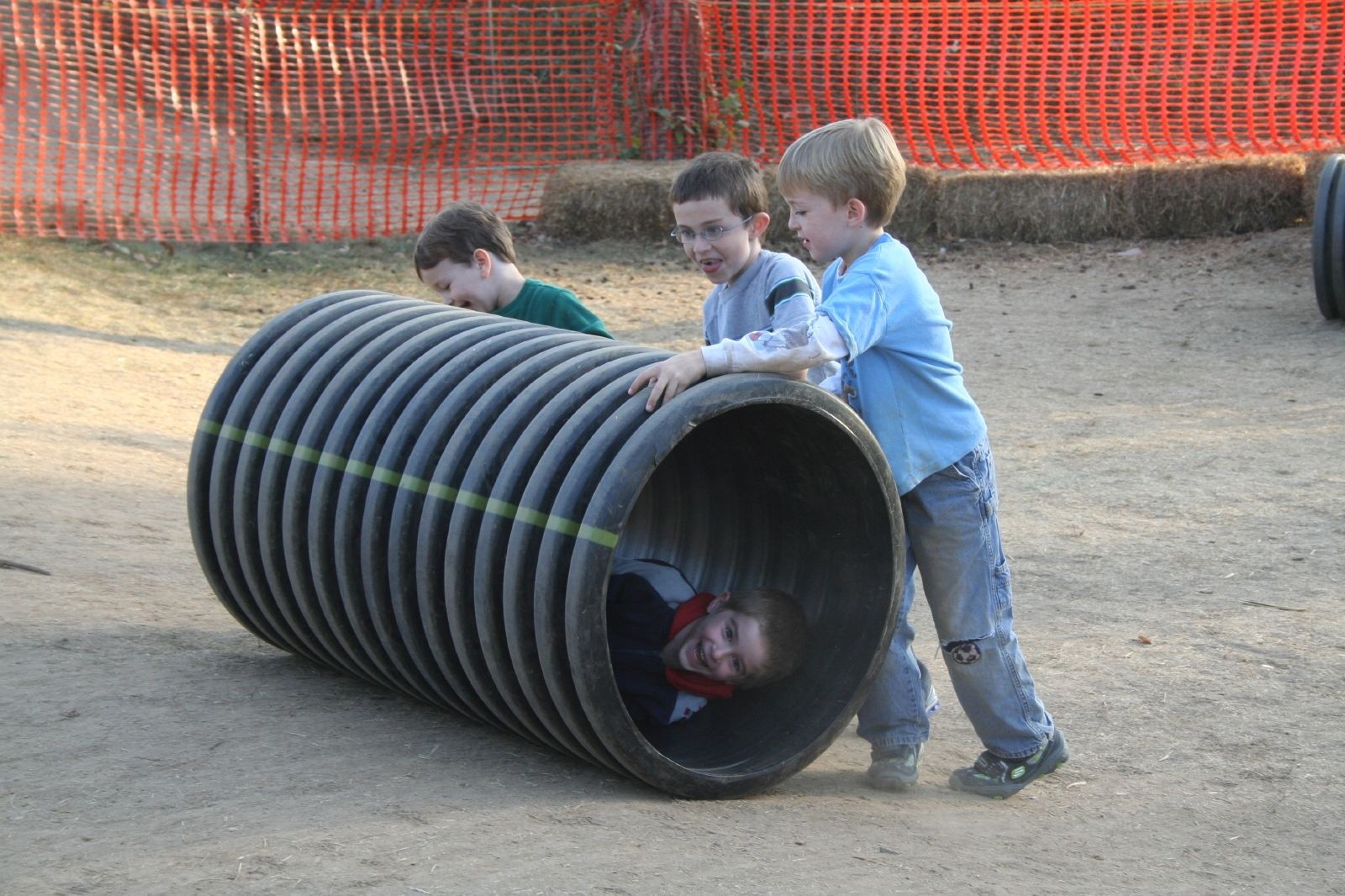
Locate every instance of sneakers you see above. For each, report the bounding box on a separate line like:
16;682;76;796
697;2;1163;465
949;725;1070;799
916;659;940;712
867;741;925;790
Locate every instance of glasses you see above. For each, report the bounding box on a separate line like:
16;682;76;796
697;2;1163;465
671;215;755;244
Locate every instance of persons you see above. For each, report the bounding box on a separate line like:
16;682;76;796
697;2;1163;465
665;150;842;399
606;559;804;733
412;203;614;340
629;117;1070;801
